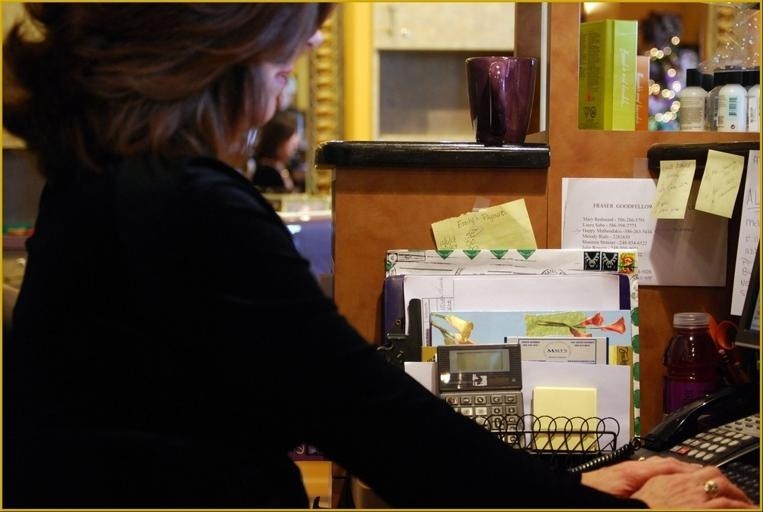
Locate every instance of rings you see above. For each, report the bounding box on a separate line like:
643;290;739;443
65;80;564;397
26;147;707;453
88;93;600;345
703;478;719;500
638;456;645;460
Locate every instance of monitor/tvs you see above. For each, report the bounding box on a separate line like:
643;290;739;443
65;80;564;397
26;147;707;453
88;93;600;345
734;239;761;349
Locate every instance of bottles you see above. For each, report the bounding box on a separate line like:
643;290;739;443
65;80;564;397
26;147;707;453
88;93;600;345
678;66;761;132
661;311;719;421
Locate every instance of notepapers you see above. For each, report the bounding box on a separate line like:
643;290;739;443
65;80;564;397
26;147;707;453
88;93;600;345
533;387;597;451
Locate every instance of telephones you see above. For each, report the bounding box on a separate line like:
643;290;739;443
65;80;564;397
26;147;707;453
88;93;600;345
644;382;759;466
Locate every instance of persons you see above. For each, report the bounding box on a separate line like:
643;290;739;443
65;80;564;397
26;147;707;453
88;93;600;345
242;72;308;193
2;3;752;510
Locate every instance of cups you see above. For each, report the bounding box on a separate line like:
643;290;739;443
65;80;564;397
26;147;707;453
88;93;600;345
464;55;539;147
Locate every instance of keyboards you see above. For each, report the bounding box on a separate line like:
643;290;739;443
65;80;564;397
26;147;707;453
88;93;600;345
716;460;760;506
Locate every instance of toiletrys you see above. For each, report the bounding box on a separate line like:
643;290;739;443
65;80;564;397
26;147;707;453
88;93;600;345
679;63;761;132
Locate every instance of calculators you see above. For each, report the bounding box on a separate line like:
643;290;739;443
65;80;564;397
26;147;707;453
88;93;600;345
437;343;527;449
